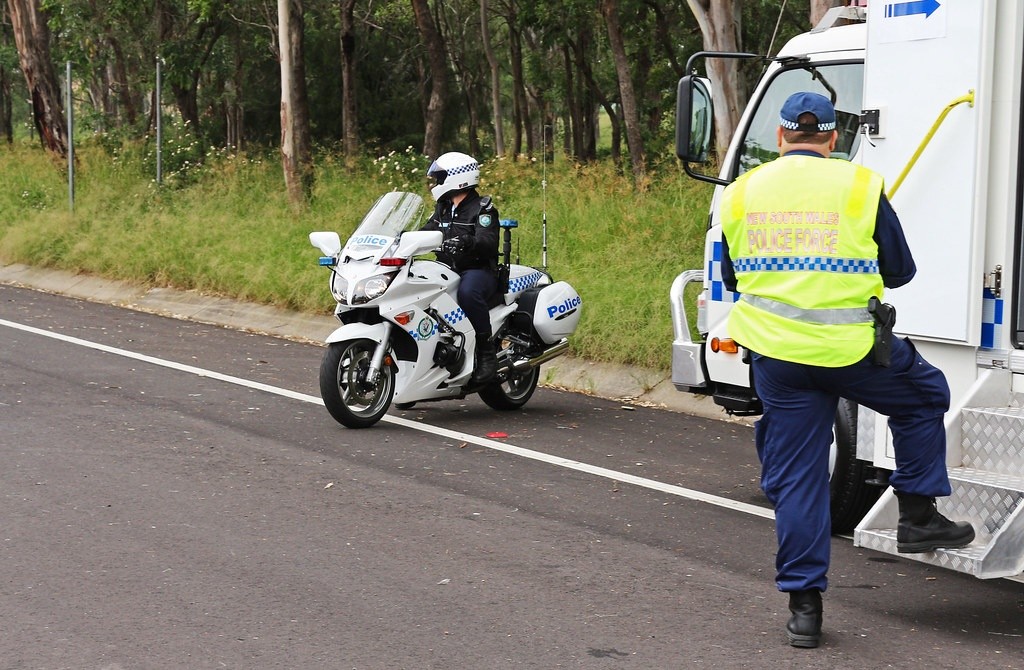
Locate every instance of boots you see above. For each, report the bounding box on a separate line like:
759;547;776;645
893;488;975;553
786;589;823;648
473;330;496;383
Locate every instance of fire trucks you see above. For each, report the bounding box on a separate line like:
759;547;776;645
666;0;1024;583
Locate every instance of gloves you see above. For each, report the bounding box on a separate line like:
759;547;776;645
442;234;473;258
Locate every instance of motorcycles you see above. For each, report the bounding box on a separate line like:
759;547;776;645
308;191;584;428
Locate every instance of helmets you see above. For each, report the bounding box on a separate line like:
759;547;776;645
426;151;480;202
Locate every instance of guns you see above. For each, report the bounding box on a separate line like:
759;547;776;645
867;294;897;368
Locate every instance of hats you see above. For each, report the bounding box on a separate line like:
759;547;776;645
779;92;837;131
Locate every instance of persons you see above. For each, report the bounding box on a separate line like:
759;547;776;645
721;93;975;650
418;152;500;394
